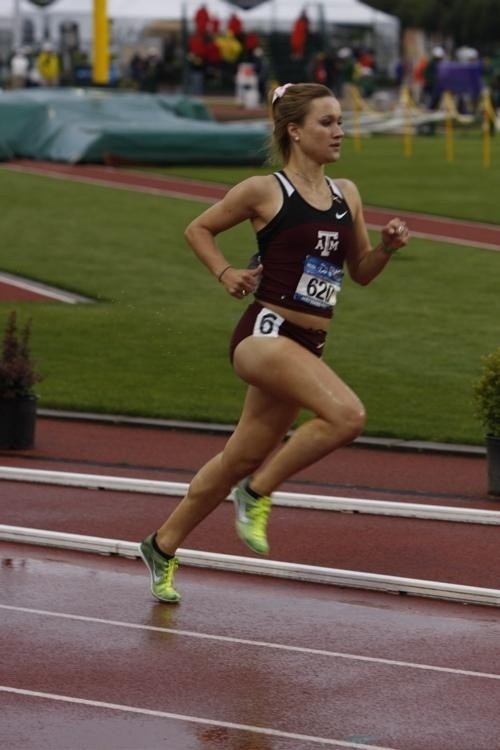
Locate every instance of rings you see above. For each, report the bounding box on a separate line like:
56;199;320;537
241;290;246;297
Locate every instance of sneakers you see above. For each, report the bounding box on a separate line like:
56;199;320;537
140;532;183;605
234;477;272;555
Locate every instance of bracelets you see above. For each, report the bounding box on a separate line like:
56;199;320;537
217;266;233;281
381;243;397;254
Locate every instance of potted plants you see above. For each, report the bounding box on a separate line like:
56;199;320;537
474;352;499;503
1;311;37;448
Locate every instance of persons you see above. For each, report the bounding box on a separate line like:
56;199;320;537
138;83;410;605
0;0;488;122
